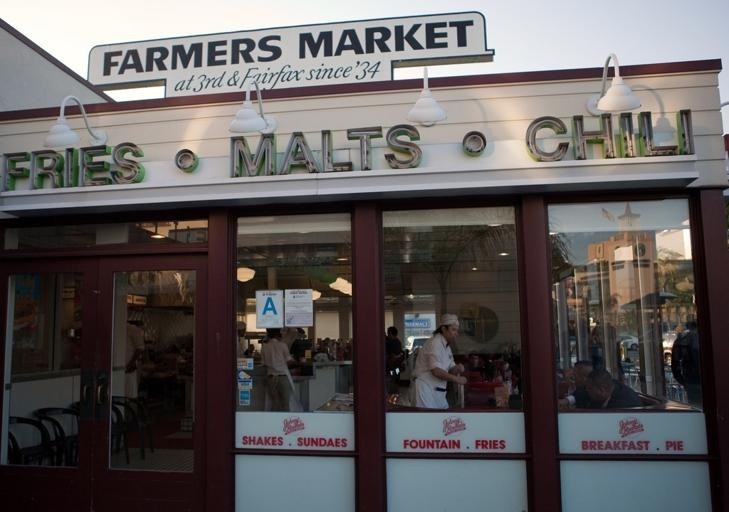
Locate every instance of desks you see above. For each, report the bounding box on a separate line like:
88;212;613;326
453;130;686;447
451;349;663;412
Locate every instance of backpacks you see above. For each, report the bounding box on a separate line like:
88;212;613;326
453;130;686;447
666;333;695;384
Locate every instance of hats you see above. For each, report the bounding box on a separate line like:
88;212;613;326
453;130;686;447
440;313;459;326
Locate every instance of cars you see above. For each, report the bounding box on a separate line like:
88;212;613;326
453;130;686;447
406;331;431;349
559;321;696;387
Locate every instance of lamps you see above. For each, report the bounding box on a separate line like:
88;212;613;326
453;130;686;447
329;275;354;297
41;95;108;150
226;78;280;136
583;50;644;118
236;264;257;284
404;65;448;128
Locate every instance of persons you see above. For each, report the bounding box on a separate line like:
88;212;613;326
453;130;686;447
237;320;307;412
386;313;524;409
558;314;703;410
125;321;185;431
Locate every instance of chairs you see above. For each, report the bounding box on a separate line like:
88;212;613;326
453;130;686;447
70;399;131;465
111;392;157;461
30;405;84;467
6;415;58;468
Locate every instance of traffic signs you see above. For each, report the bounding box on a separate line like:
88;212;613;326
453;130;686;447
402;318;432;329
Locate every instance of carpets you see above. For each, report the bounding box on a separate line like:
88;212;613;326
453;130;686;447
106;442;193;472
